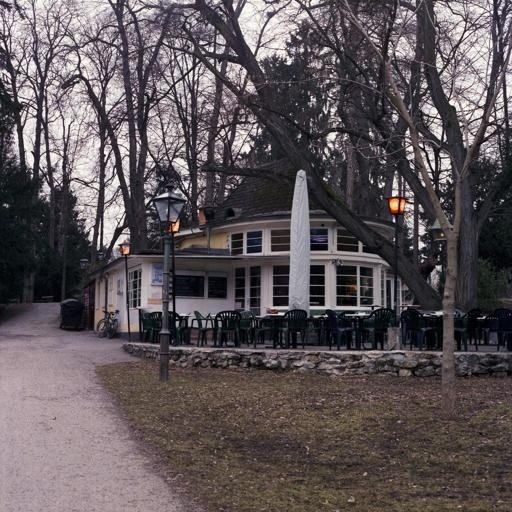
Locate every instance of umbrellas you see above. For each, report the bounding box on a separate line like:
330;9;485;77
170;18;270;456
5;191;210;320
288;170;313;318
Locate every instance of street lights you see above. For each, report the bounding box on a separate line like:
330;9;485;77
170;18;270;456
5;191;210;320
386;195;454;350
151;177;188;381
97;249;104;262
79;256;88;278
118;240;133;345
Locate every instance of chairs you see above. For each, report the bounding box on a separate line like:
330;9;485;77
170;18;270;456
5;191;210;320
141;304;512;351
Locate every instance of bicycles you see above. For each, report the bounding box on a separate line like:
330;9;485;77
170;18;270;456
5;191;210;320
95;309;119;339
